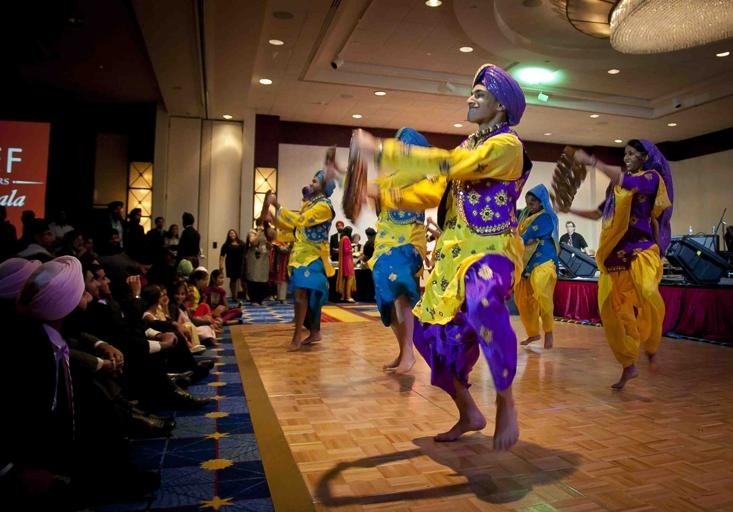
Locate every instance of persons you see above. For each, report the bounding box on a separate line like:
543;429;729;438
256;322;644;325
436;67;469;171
1;201;243;512
559;221;591;258
242;216;442;308
562;140;673;390
260;170;336;351
343;63;531;454
515;184;561;351
322;125;434;376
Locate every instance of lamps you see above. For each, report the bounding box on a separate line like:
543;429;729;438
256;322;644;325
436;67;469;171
608;0;733;56
253;166;278;230
125;162;153;236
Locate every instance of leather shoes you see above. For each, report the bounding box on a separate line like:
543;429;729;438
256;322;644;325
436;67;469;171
164;359;215;410
133;411;178;439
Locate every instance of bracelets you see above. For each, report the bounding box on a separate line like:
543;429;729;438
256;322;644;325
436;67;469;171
373;135;385;161
589;155;599;168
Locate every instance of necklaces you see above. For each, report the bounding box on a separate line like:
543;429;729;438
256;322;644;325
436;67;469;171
465;121;510;143
308;195;327;204
